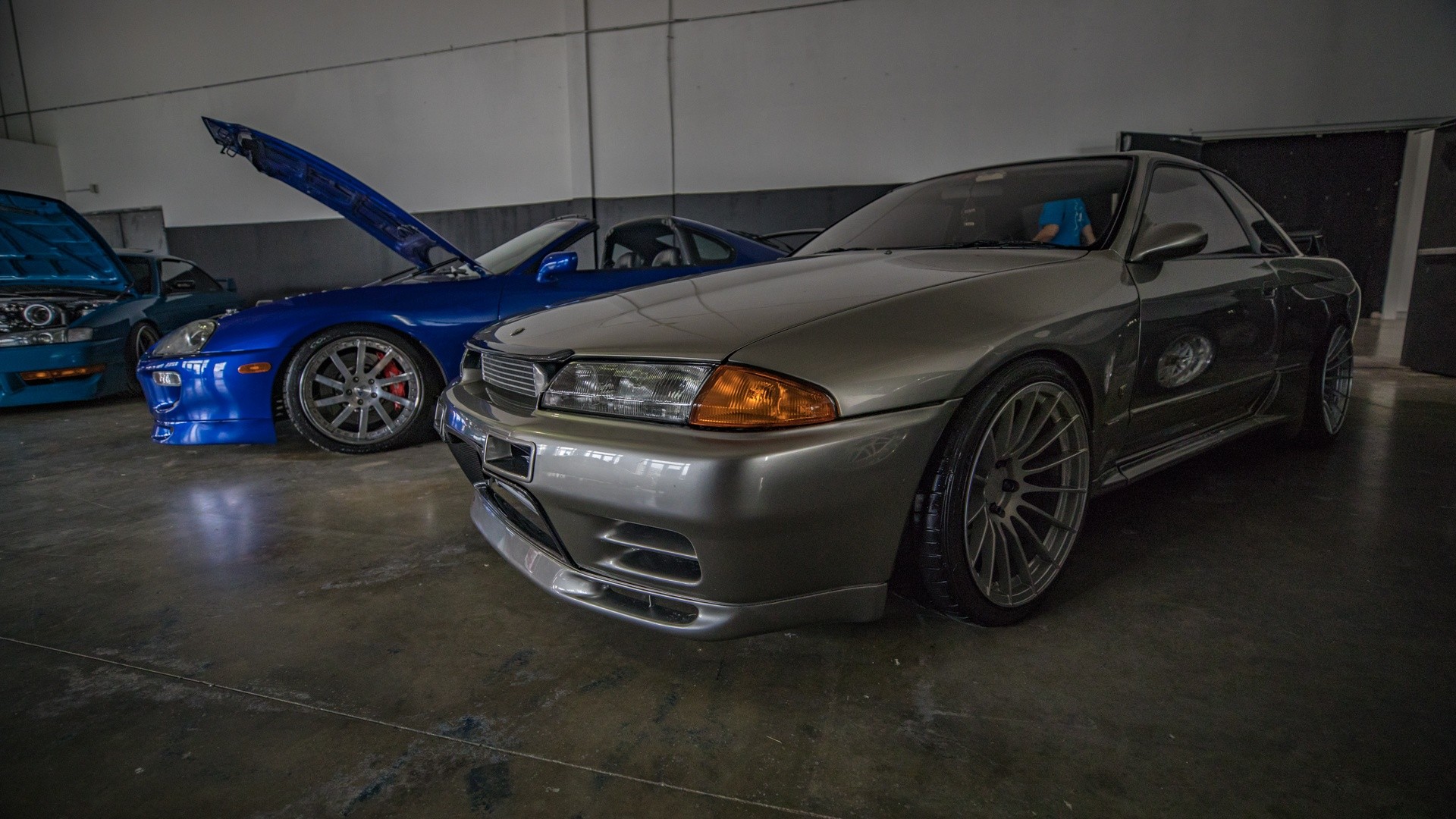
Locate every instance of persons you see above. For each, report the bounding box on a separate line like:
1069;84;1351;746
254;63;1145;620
1033;197;1096;247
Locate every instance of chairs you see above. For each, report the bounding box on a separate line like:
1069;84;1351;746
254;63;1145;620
615;251;640;268
651;248;682;267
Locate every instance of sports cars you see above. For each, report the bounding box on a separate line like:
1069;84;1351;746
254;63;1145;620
132;113;828;459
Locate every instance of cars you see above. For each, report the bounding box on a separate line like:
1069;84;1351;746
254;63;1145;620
0;187;244;409
428;147;1365;642
